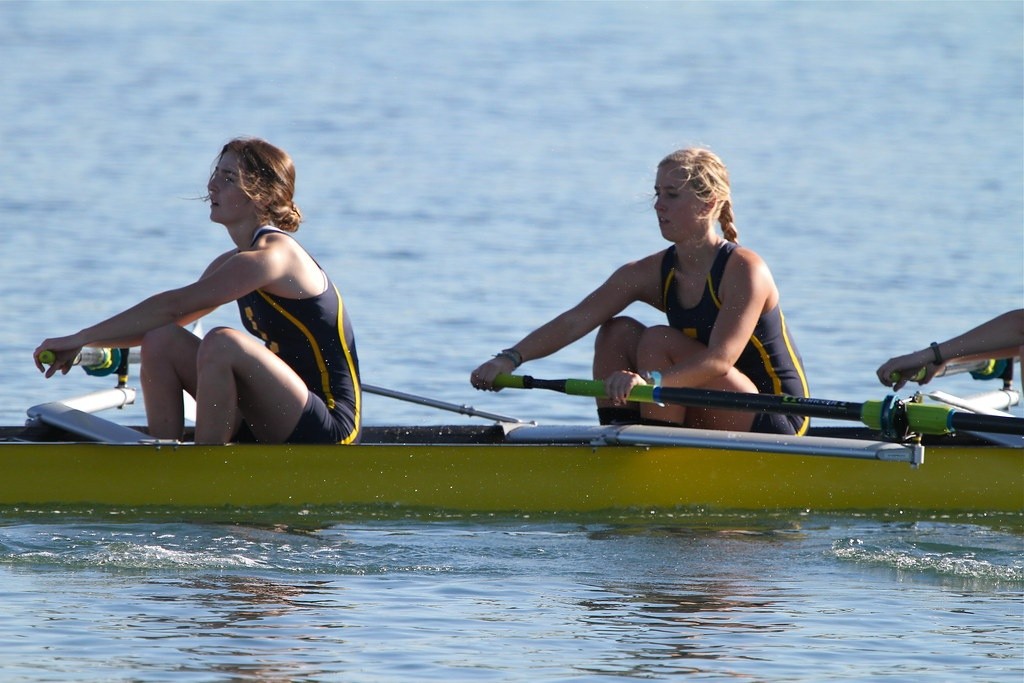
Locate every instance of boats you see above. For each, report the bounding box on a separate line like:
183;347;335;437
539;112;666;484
0;420;1024;521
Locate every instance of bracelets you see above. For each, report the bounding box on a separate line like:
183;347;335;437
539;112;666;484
642;369;664;387
497;348;522;368
930;340;943;366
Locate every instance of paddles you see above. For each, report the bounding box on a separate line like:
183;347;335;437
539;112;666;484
36;316;541;428
884;344;1023;388
485;366;1024;439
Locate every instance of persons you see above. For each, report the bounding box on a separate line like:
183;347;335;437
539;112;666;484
876;308;1024;399
470;147;812;438
35;139;363;446
